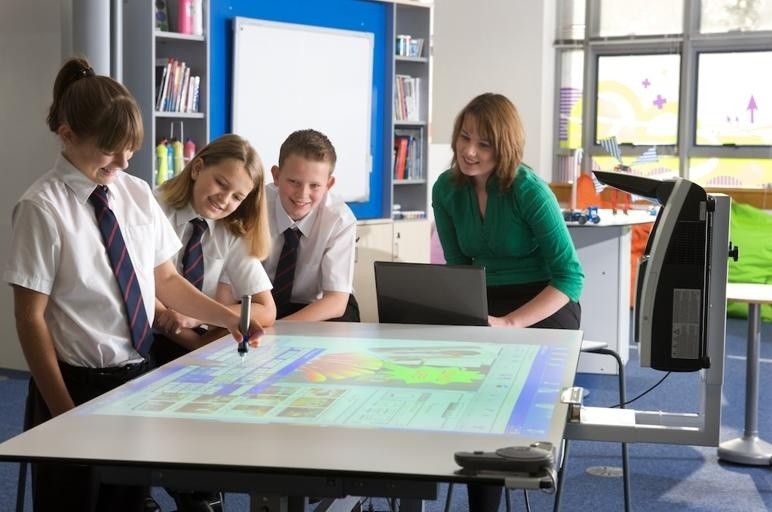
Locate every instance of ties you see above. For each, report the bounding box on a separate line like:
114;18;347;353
271;227;301;319
82;182;154;358
181;220;207;293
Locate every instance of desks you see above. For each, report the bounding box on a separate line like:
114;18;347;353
0;322;582;512
557;208;655;374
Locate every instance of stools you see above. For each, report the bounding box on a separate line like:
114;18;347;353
445;339;631;512
718;280;772;465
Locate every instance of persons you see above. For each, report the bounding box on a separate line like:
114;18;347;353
260;129;360;323
143;133;277;511
431;91;586;328
4;57;267;511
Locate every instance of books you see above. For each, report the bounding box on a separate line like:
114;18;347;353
395;34;426;180
154;0;203;112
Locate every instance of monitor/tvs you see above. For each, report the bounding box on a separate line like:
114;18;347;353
374;261;489;327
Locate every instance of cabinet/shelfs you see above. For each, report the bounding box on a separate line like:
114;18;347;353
355;222;433;321
121;0;209;192
382;0;434;221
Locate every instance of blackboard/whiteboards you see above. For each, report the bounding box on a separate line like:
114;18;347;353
231;16;375;203
